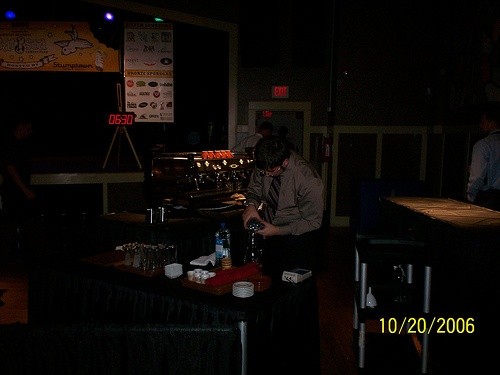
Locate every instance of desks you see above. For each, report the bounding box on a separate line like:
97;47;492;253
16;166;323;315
78;252;321;375
379;196;500;230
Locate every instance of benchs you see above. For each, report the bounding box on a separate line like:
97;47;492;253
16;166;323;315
0;321;246;375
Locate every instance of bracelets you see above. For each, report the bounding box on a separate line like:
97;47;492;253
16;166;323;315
245;203;256;207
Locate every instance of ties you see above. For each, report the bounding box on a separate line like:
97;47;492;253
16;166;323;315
264;175;281;224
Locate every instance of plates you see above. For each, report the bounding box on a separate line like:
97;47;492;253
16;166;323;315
232;281;254;298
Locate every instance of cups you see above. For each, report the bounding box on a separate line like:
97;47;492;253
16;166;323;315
187;269;216;285
202;150;234;160
123;242;164;272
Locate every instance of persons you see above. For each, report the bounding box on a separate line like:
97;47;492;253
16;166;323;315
464;103;500;321
232;122;273;153
0;118;38;273
242;135;324;373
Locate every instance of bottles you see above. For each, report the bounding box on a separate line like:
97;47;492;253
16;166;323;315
180;154;253;192
322;137;330;158
311;136;320;160
215;222;230;266
163;240;177;265
160;192;175;220
246;200;268;231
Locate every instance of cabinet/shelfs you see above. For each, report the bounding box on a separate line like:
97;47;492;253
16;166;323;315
352;234;432;373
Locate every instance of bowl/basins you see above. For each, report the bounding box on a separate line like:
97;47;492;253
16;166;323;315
249;273;271;292
